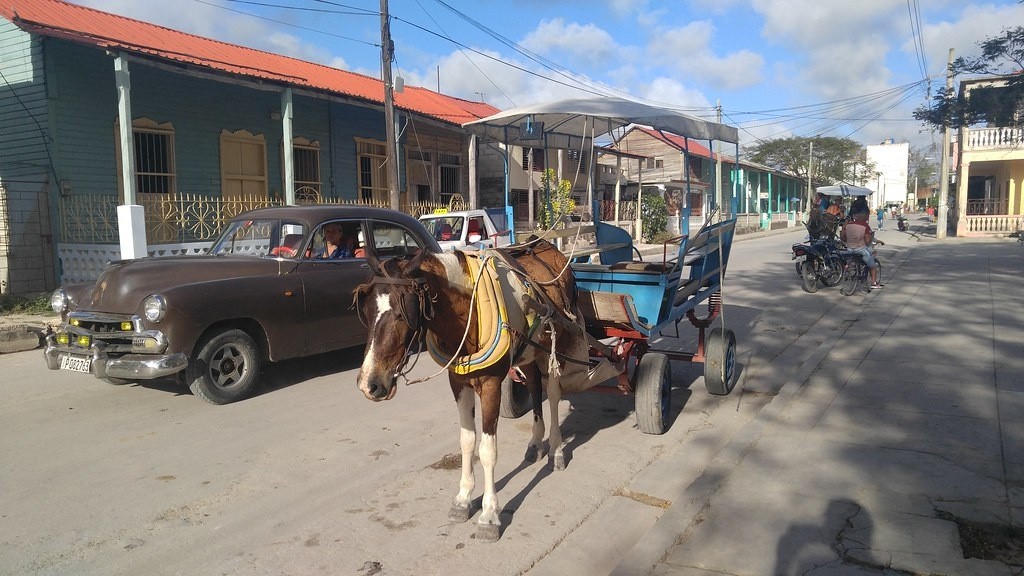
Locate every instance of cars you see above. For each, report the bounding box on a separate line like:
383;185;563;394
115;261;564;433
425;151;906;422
27;204;444;407
399;209;510;253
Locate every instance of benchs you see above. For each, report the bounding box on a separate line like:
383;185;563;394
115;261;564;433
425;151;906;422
518;220;732;269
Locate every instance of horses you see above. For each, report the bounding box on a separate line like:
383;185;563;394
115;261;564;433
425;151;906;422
806;202;838;241
352;235;581;545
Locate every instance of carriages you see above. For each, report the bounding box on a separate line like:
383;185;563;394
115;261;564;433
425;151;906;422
343;97;740;544
801;184;874;244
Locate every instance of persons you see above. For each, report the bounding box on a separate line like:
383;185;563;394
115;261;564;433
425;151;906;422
891;205;896;214
934;209;938;220
928;207;933;222
316;222;355;259
877;206;884;231
848;195;869;222
840;215;885;289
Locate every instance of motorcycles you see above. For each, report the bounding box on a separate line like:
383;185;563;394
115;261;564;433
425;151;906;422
896;215;910;232
792;231;845;293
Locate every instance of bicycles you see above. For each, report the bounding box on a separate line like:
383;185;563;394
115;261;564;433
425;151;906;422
841;241;885;295
